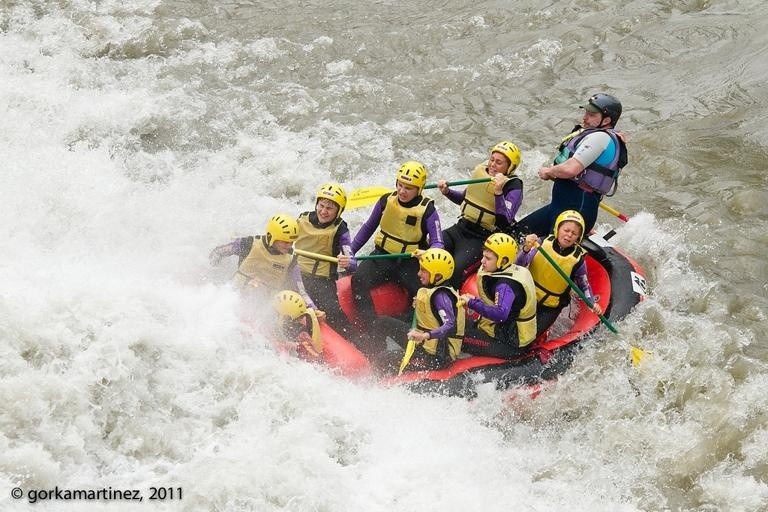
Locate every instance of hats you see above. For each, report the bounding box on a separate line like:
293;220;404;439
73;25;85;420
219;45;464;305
579;102;601;113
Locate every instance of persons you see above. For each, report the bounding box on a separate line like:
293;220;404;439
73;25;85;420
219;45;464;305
294;185;354;341
515;209;602;341
459;233;540;360
349;159;443;330
352;247;465;378
208;213;326;332
237;290;323;370
437;141;523;292
510;93;628;241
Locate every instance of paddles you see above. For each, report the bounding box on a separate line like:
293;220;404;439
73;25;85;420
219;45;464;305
344;178;496;211
529;241;650;367
398;307;416;376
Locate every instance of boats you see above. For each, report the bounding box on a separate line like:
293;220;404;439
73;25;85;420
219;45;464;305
266;231;645;404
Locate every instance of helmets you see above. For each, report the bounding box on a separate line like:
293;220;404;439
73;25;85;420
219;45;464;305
315;184;347;218
491;141;520;176
272;290;307;321
265;214;299;247
418;247;454;286
396;160;426;196
484;232;518;270
554;210;585;246
588;93;622;126
